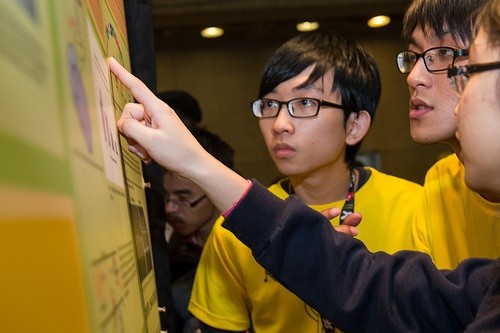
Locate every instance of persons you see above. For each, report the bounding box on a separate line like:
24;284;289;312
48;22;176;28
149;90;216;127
186;31;425;333
322;0;486;237
109;0;500;333
142;90;202;239
157;127;233;333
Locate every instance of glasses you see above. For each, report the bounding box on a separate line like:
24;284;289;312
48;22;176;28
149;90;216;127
169;195;206;211
251;97;348;120
445;61;500;98
395;46;470;74
